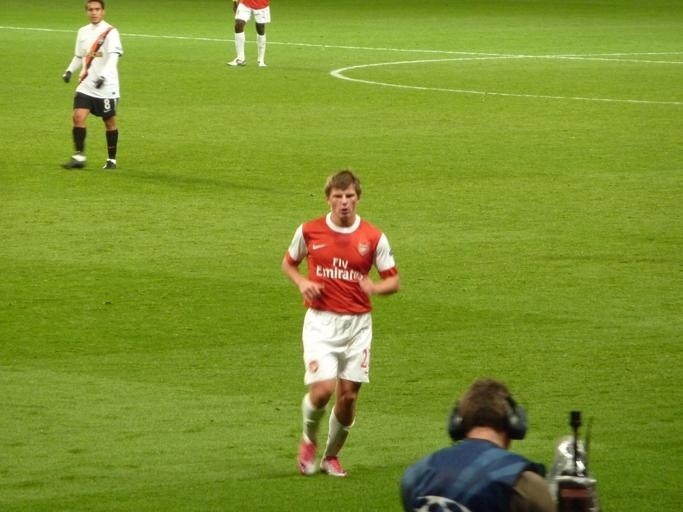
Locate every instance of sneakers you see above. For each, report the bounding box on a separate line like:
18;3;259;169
226;58;247;66
103;161;117;169
256;62;267;67
319;456;347;478
62;155;87;169
297;449;317;476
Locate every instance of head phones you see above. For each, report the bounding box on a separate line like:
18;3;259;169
449;396;526;441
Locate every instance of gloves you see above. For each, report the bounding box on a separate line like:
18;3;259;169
62;71;72;84
92;76;105;89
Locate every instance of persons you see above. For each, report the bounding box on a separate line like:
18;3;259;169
226;0;272;67
279;166;404;480
61;0;125;170
401;377;557;512
